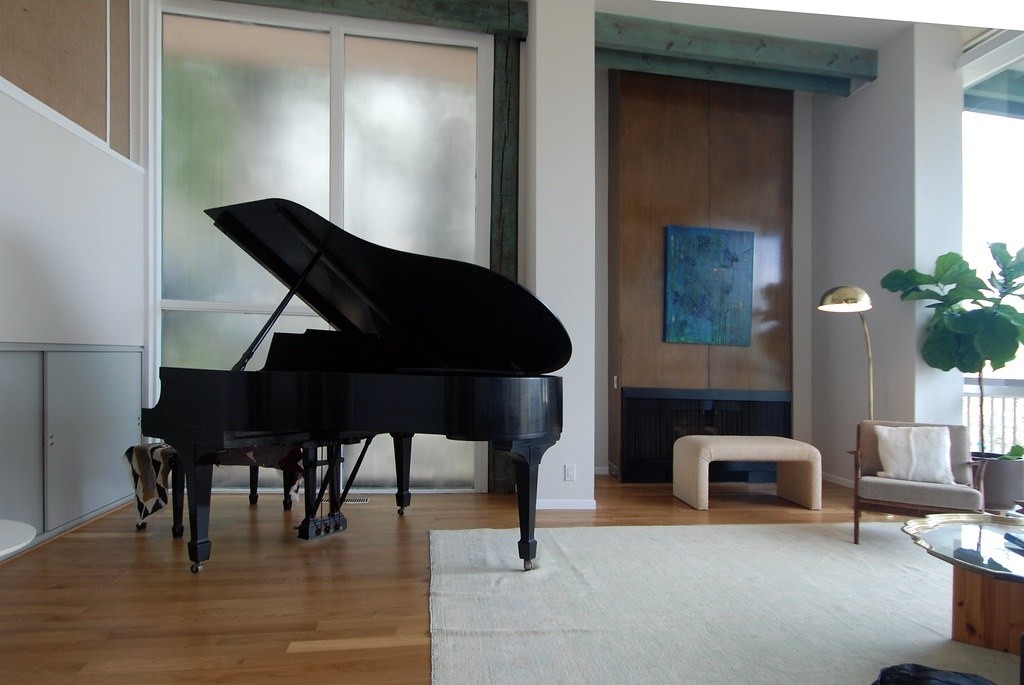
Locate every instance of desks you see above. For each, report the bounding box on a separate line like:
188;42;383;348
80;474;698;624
900;514;1024;655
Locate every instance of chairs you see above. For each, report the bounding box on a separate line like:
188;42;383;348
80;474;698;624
847;420;988;544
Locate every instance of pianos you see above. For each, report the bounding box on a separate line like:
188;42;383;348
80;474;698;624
141;197;573;572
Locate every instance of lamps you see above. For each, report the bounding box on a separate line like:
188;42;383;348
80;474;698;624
817;285;874;419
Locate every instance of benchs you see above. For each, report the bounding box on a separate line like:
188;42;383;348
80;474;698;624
122;443;304;537
673;435;822;510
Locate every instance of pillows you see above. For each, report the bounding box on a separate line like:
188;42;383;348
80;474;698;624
875;426;955;484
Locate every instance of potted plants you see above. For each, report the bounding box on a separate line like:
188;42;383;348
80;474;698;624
881;242;1024;511
